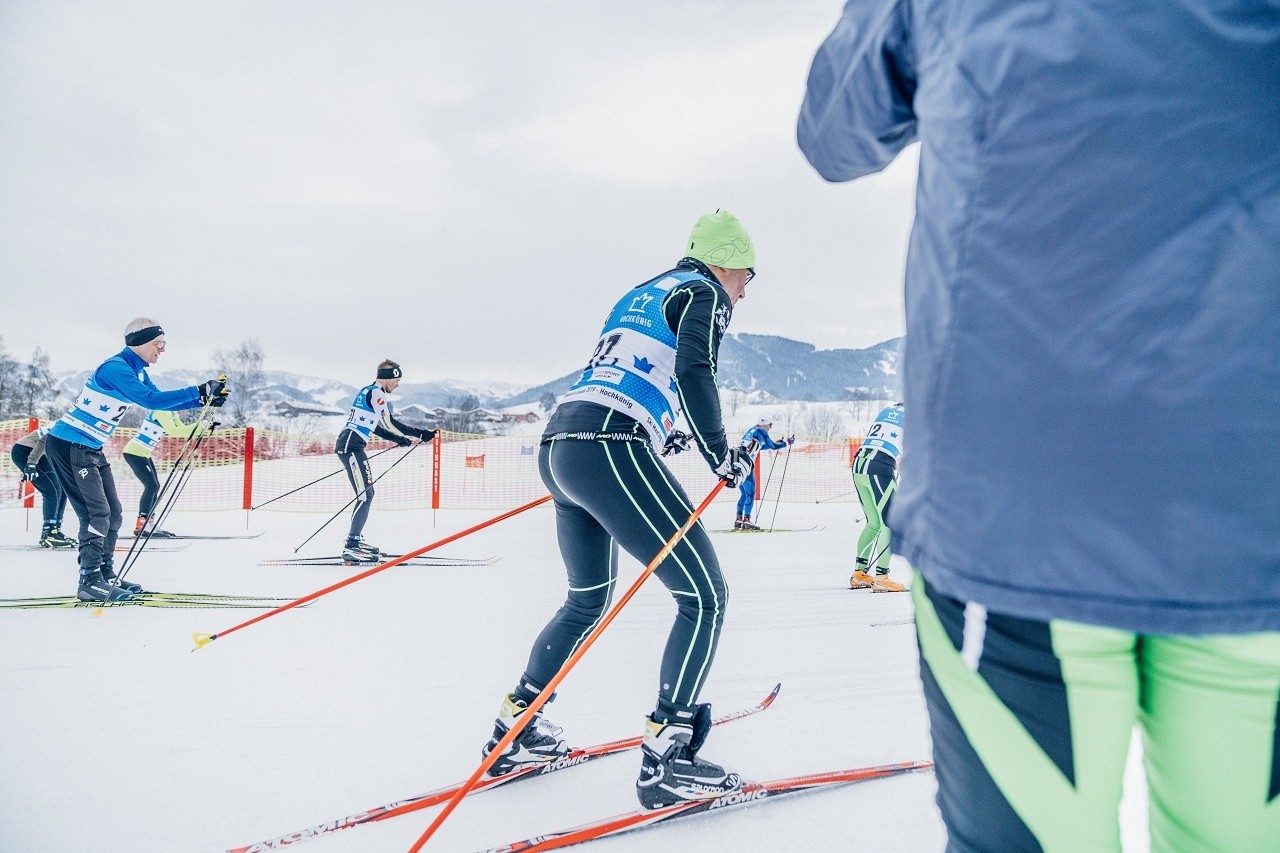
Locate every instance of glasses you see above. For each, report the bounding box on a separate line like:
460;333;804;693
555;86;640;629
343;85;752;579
745;267;756;286
149;340;166;350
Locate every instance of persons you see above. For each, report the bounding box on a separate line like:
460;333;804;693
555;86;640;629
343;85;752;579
44;318;231;601
481;210;755;810
335;360;435;562
735;416;795;530
850;403;905;592
11;417;77;548
797;0;1280;853
123;410;221;537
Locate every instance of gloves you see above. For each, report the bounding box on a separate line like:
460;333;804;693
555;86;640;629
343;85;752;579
399;437;412;448
198;379;227;399
200;392;230;407
204;420;220;432
660;429;691;457
714;445;755;489
21;463;39;482
419;428;435;442
788;437;795;444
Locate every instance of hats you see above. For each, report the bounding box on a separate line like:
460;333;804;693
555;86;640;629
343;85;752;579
758;412;772;424
686;209;756;269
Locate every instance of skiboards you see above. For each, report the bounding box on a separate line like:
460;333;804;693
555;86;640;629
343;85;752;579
0;519;192;553
1;567;321;610
253;535;504;567
224;680;937;853
117;513;269;539
705;517;827;534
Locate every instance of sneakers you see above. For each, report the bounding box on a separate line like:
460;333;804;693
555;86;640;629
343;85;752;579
101;565;142;594
850;571;876;589
343;546;381;563
57;526;77;544
634;702;746;809
871;575;905;592
481;692;571;777
734;515;761;531
37;523;73;548
133;517;175;538
359;542;386;556
77;570;134;601
